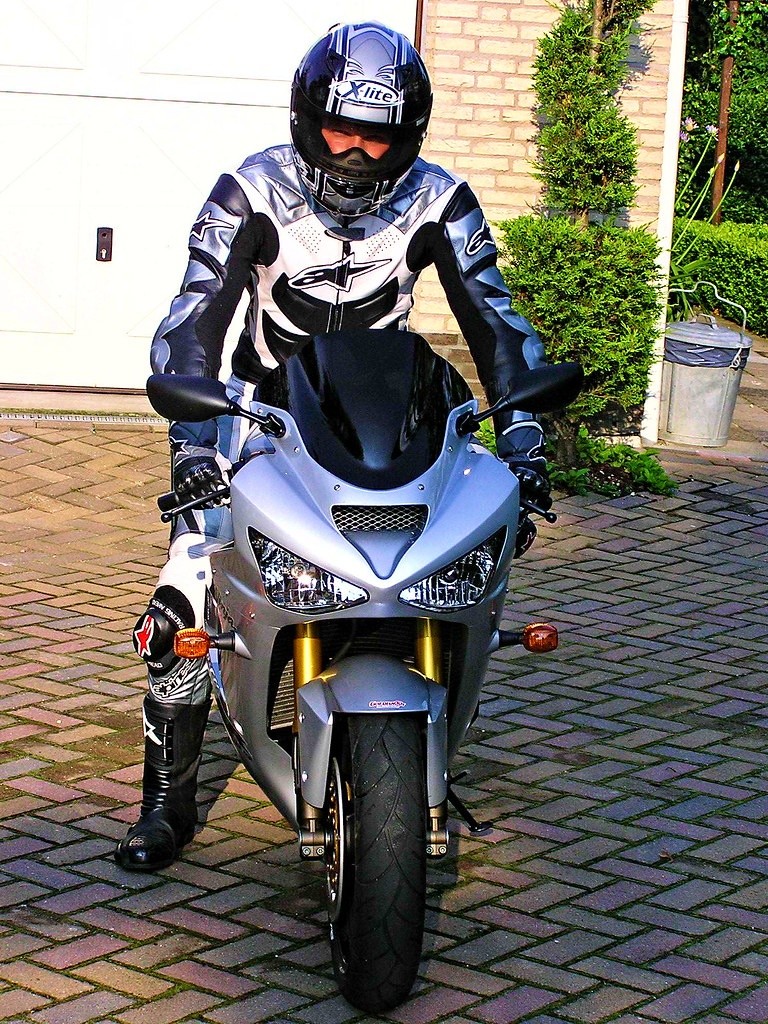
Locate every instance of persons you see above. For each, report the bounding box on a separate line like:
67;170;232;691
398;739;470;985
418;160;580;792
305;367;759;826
116;25;553;872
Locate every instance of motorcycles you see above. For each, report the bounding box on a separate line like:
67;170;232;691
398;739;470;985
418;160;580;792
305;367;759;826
146;327;589;1015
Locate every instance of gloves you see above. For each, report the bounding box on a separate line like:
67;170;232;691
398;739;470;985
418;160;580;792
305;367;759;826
494;420;552;503
167;420;232;510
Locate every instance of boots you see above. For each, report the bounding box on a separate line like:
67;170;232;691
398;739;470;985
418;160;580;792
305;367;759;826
113;694;214;874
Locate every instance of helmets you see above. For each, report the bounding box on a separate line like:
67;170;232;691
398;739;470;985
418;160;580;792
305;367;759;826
290;19;433;220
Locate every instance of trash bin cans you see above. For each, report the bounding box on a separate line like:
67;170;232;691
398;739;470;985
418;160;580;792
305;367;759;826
659;314;753;448
